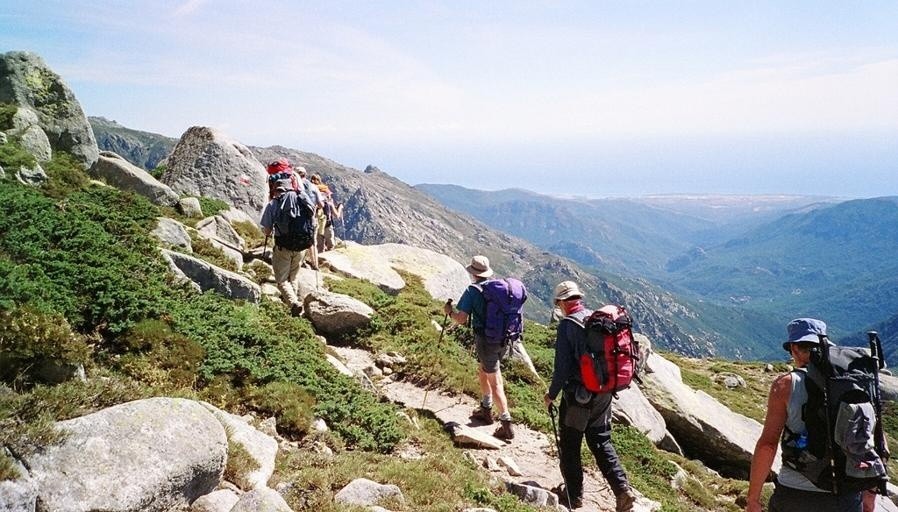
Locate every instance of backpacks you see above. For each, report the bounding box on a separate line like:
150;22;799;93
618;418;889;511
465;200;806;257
276;191;316;251
470;277;528;345
781;344;890;495
564;305;639;394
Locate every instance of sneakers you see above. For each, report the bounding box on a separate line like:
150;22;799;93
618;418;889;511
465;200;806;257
494;419;517;439
291;304;302;317
615;489;636;512
551;487;583;509
473;403;494;425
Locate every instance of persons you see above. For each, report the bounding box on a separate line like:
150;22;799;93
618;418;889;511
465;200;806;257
748;318;876;512
444;256;514;439
260;163;344;317
544;281;636;512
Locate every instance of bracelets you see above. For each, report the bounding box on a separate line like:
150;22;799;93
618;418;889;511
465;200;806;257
448;311;453;317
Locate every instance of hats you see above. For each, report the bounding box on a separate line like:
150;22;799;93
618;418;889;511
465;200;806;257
465;256;494;278
553;281;584;305
783;318;837;351
273;179;295;192
